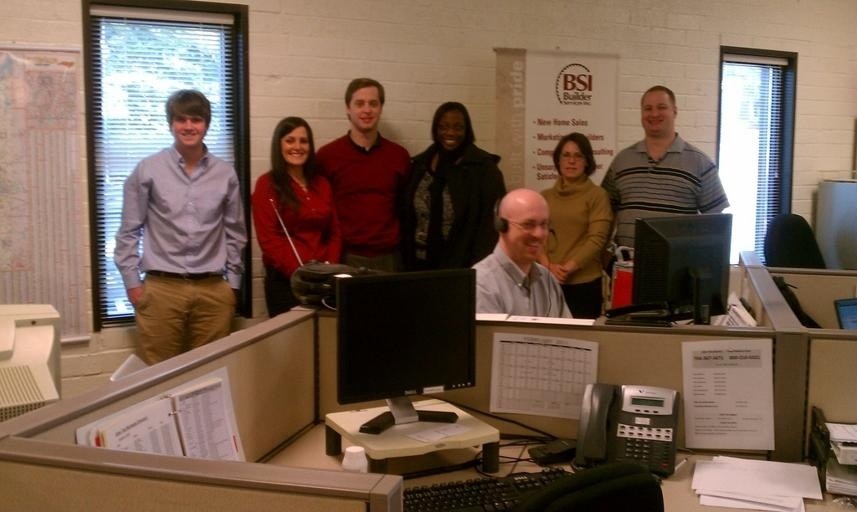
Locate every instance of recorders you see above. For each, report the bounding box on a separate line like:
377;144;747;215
291;259;374;305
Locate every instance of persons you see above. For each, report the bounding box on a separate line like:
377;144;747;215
528;131;613;320
315;78;412;277
253;116;342;317
115;89;249;373
600;85;728;258
407;101;508;281
466;189;575;321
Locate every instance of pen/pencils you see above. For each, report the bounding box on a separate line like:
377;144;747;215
675;457;687;470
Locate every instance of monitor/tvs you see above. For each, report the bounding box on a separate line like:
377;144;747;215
834;297;857;329
632;212;734;325
334;265;478;434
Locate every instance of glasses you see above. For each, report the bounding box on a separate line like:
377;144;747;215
560;149;588;161
511;217;551;231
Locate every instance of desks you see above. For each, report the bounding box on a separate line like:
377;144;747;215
259;418;857;511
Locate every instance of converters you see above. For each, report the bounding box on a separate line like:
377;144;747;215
527;435;575;464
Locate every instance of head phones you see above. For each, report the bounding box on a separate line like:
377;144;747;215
491;196;508;235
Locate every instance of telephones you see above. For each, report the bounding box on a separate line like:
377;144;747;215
576;384;680;478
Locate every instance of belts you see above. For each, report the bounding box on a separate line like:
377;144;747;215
143;267;226;282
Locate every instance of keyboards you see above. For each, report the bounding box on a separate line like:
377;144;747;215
403;469;572;511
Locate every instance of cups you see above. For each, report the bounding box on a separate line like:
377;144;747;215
343;446;367;475
